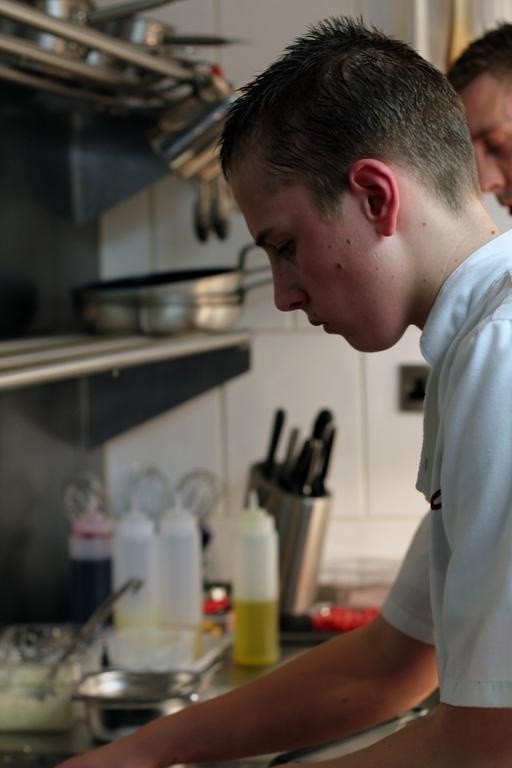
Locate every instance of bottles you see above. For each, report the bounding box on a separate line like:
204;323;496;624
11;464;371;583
67;491;280;667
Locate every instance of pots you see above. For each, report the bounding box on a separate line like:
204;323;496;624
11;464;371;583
2;0;240;75
74;241;275;335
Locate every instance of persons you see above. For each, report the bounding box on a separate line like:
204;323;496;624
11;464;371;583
62;14;512;768
444;19;511;216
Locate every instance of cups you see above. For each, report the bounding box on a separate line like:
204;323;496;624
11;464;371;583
145;74;252;181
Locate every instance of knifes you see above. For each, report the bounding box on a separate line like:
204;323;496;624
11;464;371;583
264;405;339;499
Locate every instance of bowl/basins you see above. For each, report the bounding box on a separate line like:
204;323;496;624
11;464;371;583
1;620;201;747
